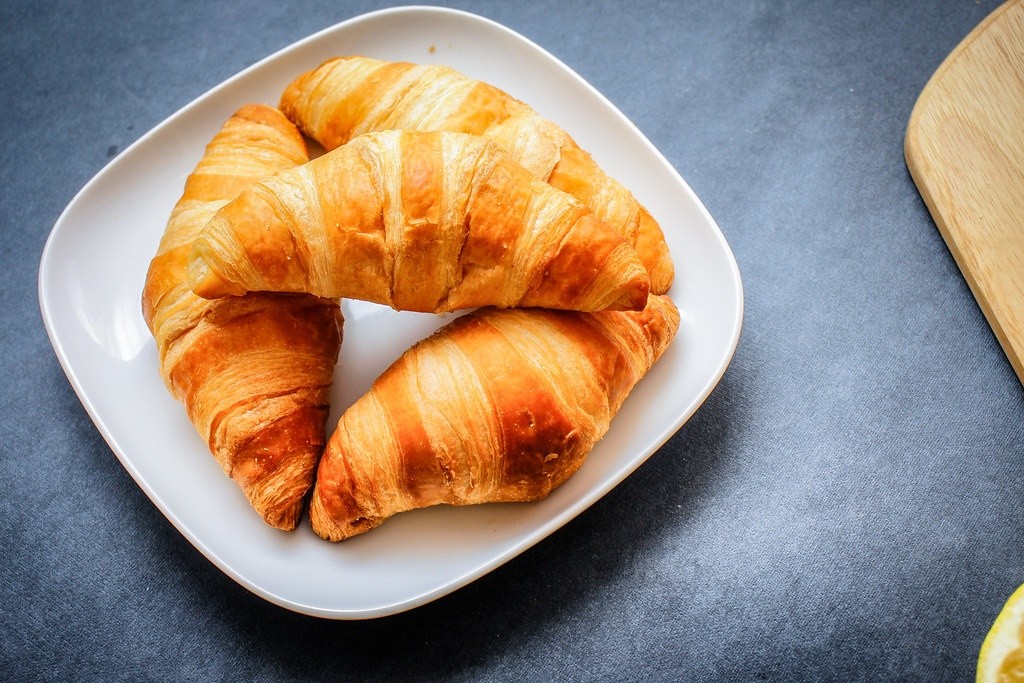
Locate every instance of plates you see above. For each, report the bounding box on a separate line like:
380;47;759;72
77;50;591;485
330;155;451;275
39;6;745;621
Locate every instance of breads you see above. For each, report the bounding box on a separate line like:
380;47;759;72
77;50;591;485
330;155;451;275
141;105;345;531
187;126;650;313
306;290;682;543
274;55;674;298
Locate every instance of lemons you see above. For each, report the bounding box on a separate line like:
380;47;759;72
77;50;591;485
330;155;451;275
976;583;1024;683
